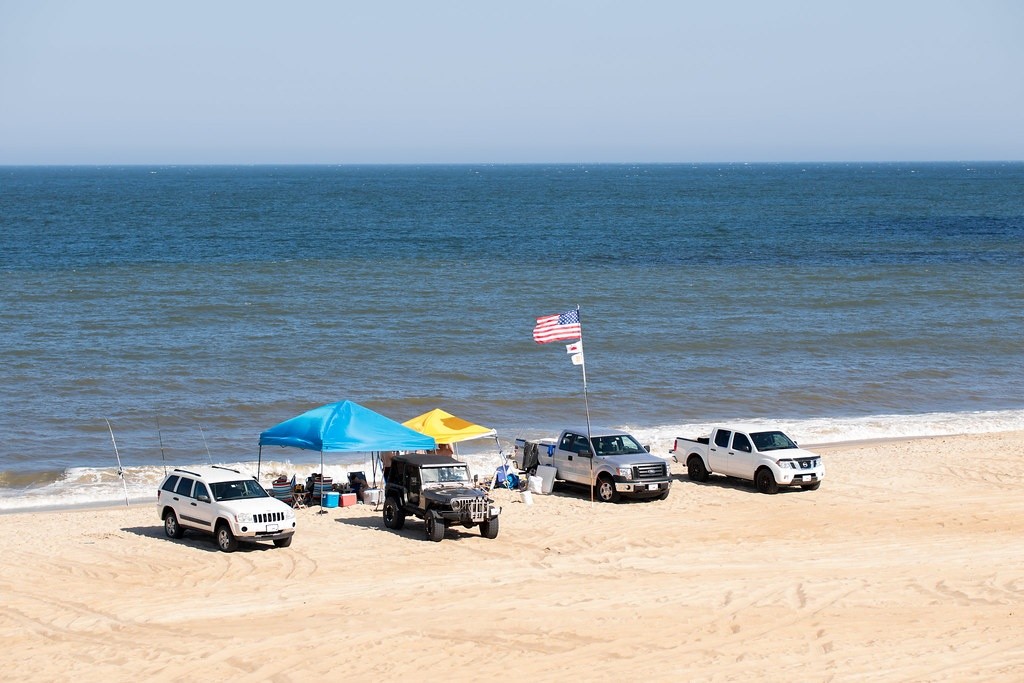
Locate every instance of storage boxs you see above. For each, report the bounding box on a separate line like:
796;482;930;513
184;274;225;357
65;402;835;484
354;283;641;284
363;490;384;505
341;493;357;507
322;491;340;508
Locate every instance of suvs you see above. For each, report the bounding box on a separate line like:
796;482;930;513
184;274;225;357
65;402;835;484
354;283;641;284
382;454;502;543
156;465;297;553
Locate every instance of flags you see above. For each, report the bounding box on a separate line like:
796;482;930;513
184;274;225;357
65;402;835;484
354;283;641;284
532;308;582;346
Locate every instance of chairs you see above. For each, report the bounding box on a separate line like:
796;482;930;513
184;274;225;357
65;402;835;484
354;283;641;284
601;438;618;451
310;477;332;506
347;471;377;500
273;480;293;507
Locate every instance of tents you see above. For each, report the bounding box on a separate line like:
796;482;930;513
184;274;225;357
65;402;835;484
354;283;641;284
250;396;438;517
399;406;513;500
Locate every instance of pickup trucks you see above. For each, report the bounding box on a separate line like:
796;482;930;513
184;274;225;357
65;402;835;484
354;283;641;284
673;427;826;495
513;426;673;503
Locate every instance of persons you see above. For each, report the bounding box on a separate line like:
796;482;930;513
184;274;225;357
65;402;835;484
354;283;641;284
376;443;454;483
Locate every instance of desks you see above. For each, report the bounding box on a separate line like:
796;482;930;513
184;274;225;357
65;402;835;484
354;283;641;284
335;486;355;493
288;492;310;509
